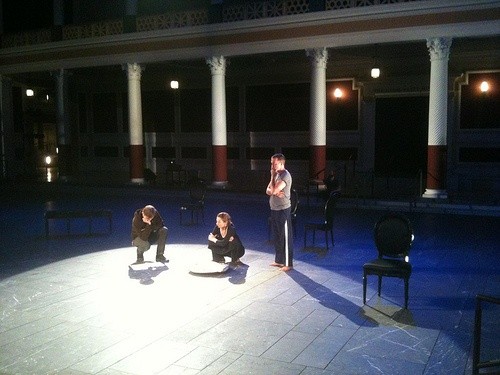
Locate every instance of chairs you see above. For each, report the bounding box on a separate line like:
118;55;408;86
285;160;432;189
362;211;415;309
267;188;300;241
176;178;208;225
303;192;338;251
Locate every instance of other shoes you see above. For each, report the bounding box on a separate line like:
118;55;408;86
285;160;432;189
137;250;144;261
156;255;169;262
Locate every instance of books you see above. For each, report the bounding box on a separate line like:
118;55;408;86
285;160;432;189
130;262;166;270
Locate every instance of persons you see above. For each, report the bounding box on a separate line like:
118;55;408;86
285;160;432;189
131;205;169;262
266;153;294;271
207;211;245;265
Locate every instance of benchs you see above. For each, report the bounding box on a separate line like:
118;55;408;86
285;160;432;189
45;210;112;240
